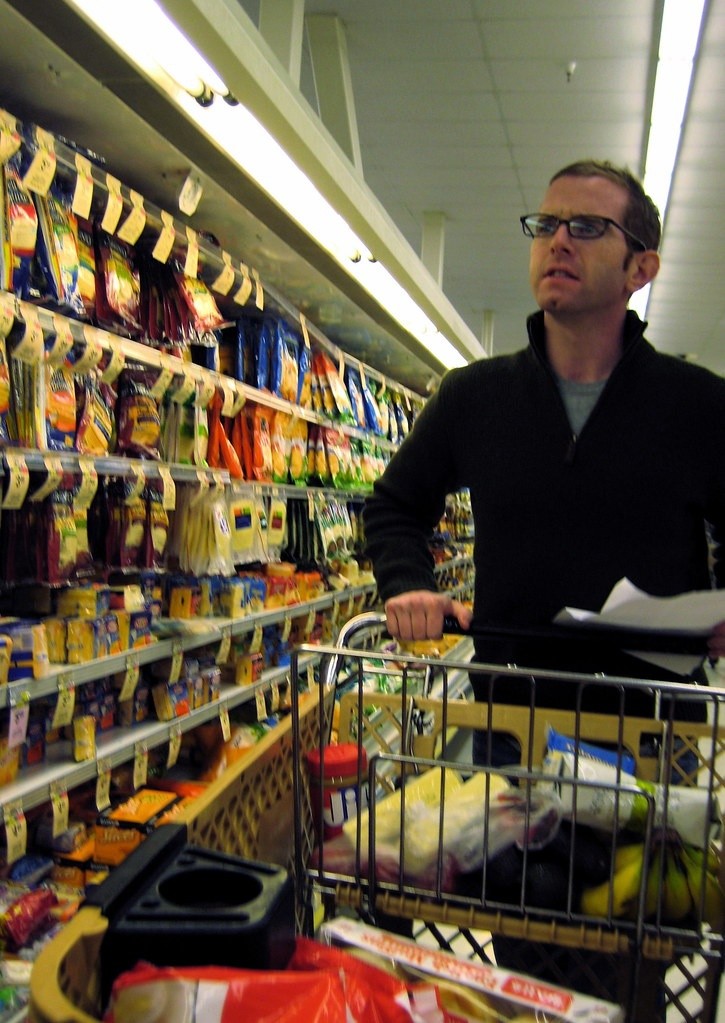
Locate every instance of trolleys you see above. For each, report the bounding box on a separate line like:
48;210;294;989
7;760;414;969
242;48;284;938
27;609;724;1023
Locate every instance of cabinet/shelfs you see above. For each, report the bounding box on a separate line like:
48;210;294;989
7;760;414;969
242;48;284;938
0;110;476;1022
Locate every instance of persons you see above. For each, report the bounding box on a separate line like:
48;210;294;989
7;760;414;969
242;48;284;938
362;164;725;1023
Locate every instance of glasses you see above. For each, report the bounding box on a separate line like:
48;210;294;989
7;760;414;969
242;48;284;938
520;213;647;249
